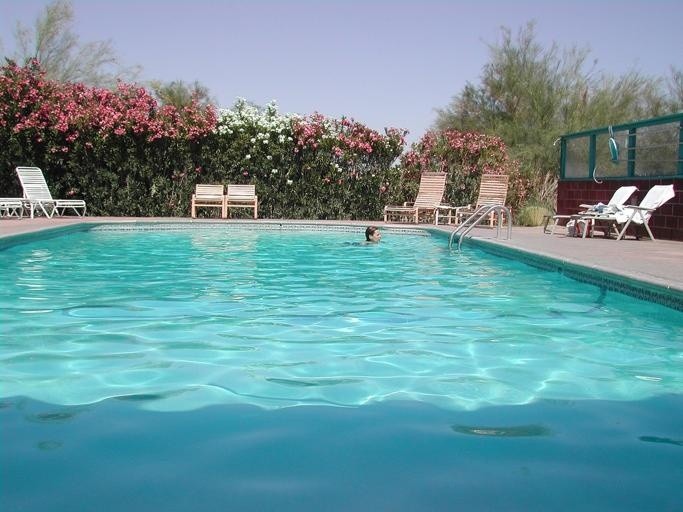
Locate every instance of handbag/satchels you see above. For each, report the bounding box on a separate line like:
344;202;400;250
566;219;592;237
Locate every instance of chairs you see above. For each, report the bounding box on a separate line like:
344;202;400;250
384;171;510;228
191;183;259;219
0;166;87;220
544;183;677;241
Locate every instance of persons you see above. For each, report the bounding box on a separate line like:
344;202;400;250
362;226;382;243
